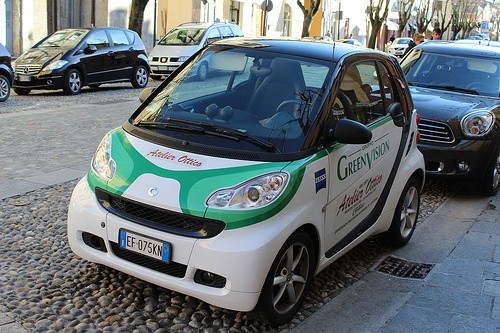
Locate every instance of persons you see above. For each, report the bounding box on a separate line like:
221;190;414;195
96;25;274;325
405;34;423;56
431;28;441;41
347;33;354;39
326;30;330;37
390;34;395;42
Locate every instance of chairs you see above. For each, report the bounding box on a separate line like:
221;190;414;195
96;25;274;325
313;64;368;135
244;58;306;121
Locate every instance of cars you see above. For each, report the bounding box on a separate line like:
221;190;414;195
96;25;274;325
0;42;14;102
469;34;490;40
11;27;152;95
388;37;414;55
64;36;431;325
370;39;500;196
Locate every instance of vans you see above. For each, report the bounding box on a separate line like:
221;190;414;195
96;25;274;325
148;20;248;81
